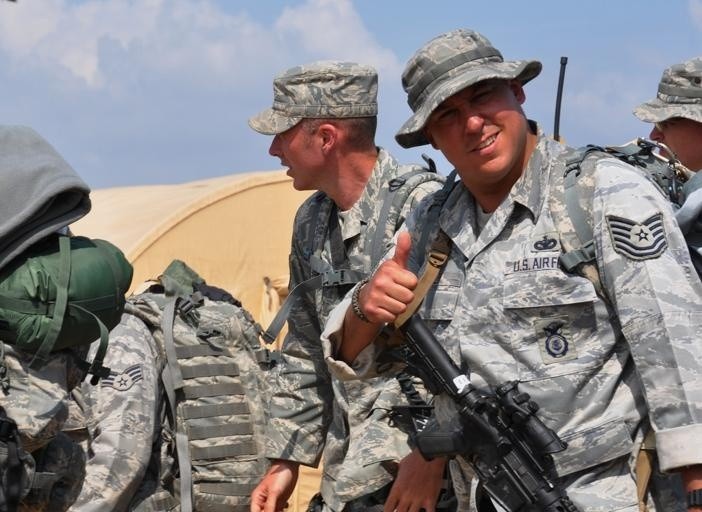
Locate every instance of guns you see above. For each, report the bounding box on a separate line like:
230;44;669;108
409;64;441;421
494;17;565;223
376;313;581;512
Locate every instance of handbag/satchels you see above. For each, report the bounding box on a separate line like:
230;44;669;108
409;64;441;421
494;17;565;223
0;237;134;353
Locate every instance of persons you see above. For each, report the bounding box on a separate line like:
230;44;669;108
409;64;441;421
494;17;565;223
317;25;702;512
249;58;446;512
63;298;180;512
630;56;701;174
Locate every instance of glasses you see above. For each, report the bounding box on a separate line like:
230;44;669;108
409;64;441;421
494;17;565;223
655;121;680;135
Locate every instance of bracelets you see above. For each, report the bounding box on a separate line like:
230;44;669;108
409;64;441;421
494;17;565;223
352;277;369;323
685;487;702;509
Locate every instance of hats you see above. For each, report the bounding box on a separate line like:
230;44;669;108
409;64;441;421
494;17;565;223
393;28;543;150
632;56;702;129
247;59;380;137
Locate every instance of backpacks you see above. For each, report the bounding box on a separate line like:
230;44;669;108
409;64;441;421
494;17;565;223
126;260;291;511
